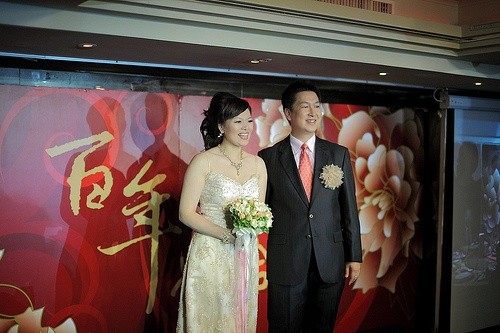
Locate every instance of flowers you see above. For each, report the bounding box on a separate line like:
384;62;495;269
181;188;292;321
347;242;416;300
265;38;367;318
254;99;343;150
225;196;274;236
337;107;423;295
318;164;344;190
0;250;78;333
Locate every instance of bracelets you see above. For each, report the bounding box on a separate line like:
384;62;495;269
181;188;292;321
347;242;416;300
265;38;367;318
223;229;230;244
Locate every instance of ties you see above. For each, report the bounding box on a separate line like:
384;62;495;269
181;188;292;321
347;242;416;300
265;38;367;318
298;143;313;202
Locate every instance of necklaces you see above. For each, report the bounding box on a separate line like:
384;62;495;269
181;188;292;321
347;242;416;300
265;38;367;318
218;144;243;176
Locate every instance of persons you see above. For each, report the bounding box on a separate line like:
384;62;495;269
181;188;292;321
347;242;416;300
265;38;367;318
257;83;363;333
176;91;268;333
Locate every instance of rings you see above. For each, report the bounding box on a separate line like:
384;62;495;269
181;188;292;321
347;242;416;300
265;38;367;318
355;276;358;277
